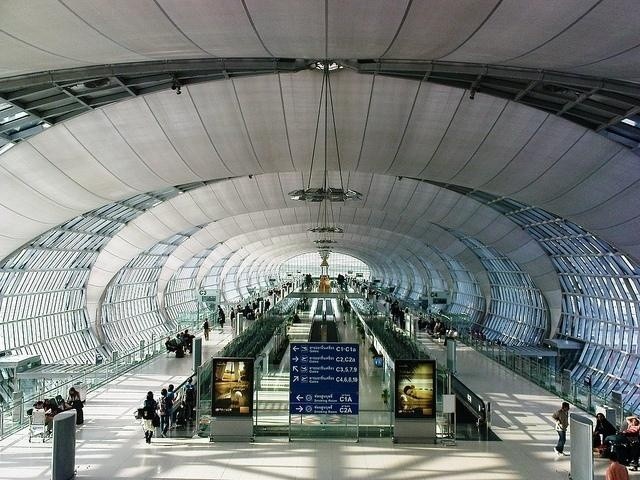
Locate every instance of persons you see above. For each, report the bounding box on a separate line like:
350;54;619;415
217;273;290;334
352;279;409;329
593;413;640;443
418;312;458;346
165;330;195;358
33;387;83;434
606;462;628;480
551;402;569;456
404;386;432;408
142;378;195;444
203;318;209;340
468;330;507;346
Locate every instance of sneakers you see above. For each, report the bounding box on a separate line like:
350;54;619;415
554;447;565;457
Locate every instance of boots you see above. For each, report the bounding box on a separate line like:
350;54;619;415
146;431;153;443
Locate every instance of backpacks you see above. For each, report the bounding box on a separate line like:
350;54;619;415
186;389;193;398
204;321;208;329
143;405;154;420
